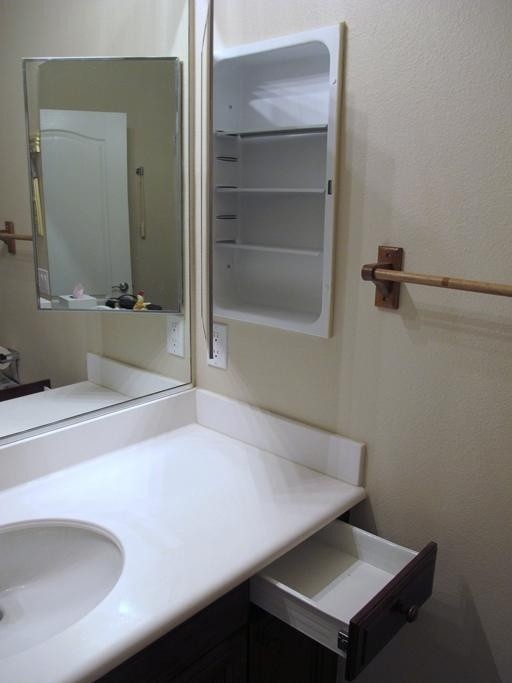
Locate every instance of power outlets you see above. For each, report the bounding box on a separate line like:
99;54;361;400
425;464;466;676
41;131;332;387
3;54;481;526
205;321;227;367
165;311;186;358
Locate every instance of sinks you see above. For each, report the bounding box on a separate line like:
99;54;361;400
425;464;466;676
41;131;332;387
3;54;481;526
0;516;127;660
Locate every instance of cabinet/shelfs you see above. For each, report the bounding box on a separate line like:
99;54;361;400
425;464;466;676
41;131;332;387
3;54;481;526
102;511;434;681
214;127;325;256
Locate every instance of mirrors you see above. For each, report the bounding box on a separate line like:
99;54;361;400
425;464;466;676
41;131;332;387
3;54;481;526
20;54;186;310
0;0;192;442
